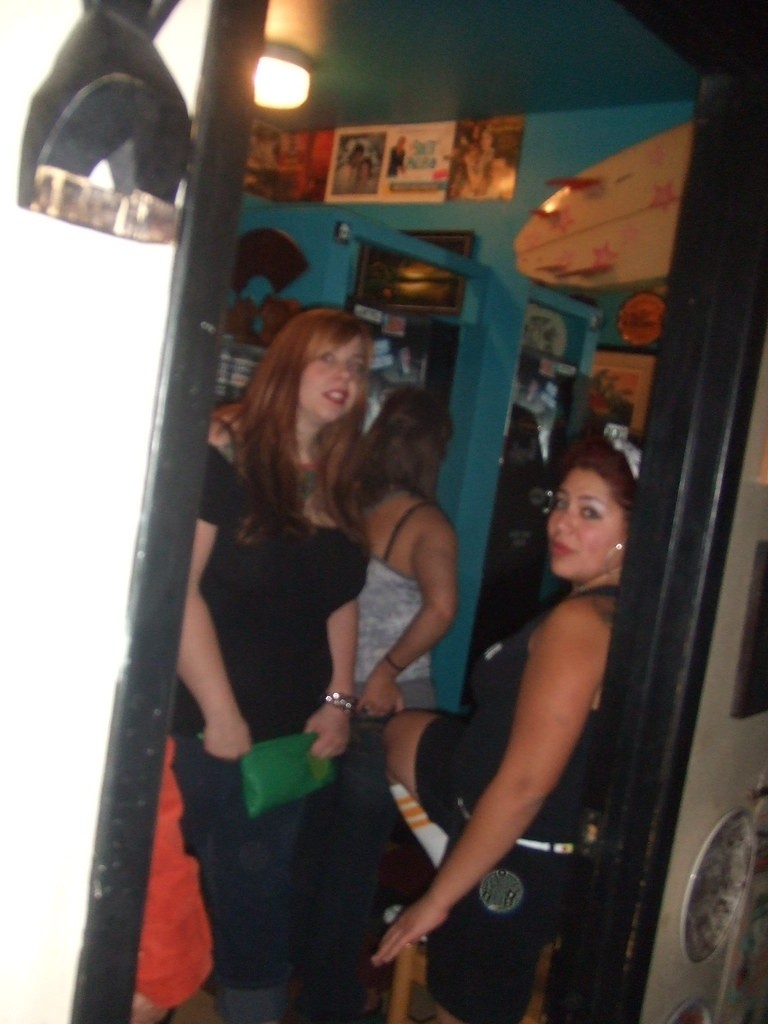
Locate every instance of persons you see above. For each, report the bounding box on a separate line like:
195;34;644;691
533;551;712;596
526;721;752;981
463;125;495;194
350;385;458;717
386;135;407;178
128;306;375;1024
459;401;553;706
290;420;642;1024
334;144;377;193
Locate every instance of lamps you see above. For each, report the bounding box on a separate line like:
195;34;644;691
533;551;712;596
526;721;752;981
253;42;319;112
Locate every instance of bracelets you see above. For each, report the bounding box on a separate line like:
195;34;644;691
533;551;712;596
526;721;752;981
320;693;355;713
386;655;405;673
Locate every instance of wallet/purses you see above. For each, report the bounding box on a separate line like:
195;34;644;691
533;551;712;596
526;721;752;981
239;731;335;819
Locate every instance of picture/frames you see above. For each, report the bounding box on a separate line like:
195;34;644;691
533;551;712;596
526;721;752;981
731;541;768;720
355;229;475;316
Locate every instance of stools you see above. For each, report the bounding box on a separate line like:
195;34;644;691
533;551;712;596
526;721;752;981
386;931;563;1024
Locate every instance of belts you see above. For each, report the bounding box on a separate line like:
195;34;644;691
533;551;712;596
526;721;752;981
455;796;594;855
